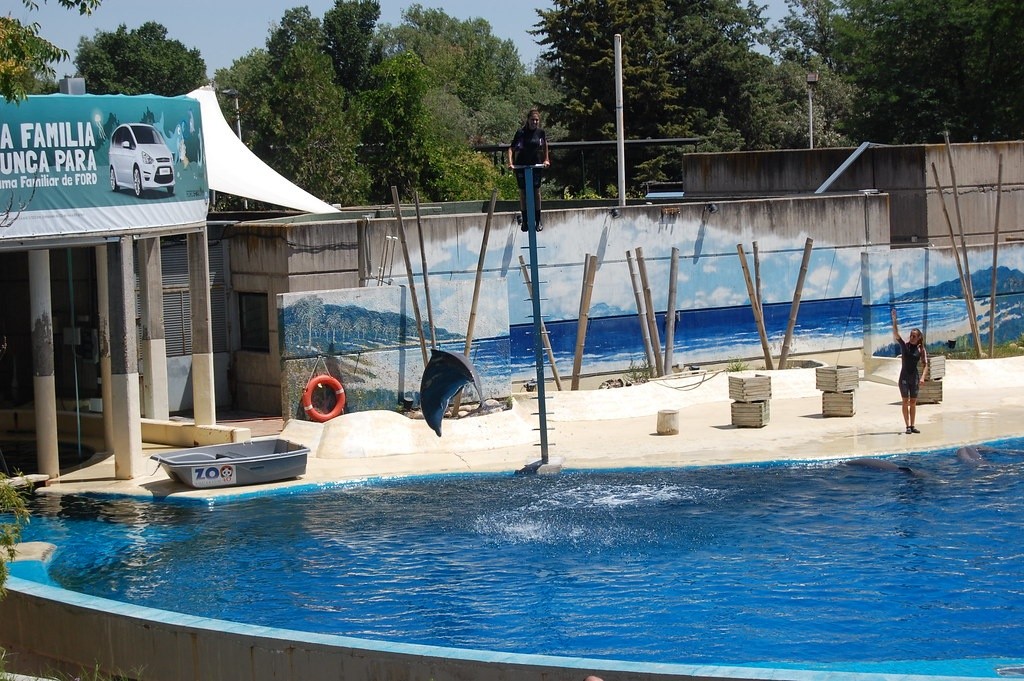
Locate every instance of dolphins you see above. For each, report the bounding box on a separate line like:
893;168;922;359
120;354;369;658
419;347;483;437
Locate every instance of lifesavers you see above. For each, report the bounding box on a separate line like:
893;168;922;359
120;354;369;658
302;375;346;422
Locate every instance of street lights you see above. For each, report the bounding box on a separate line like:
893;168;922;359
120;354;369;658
806;70;819;149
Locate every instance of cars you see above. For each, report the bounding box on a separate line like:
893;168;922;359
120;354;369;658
109;123;175;199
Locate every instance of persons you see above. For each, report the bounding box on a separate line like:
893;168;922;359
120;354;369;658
891;309;928;434
508;109;550;232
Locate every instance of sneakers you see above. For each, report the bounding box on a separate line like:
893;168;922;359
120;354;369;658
907;426;919;432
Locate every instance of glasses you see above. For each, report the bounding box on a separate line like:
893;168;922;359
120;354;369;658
910;335;919;338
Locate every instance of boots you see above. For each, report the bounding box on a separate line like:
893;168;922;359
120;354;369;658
536;210;542;232
520;210;528;231
